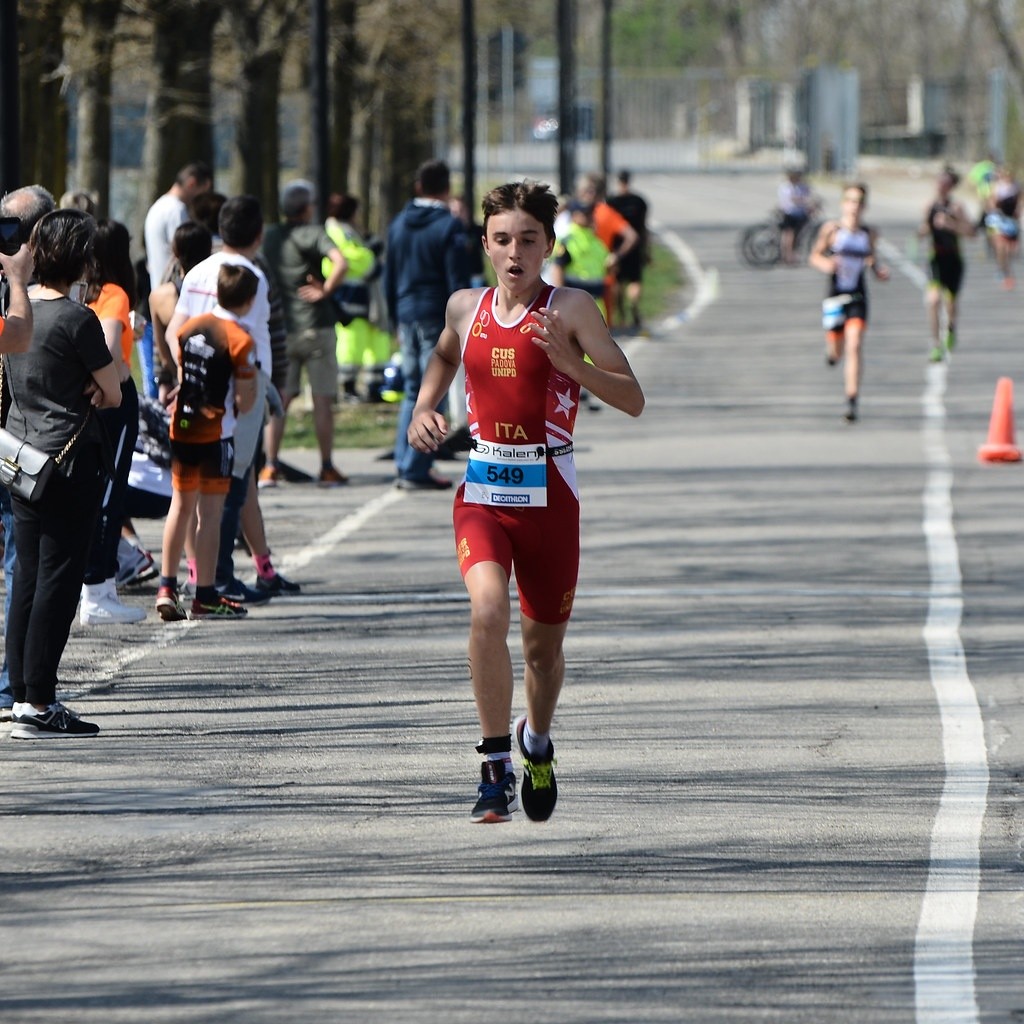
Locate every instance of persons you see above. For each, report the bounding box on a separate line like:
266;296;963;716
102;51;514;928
969;158;1020;291
810;180;890;421
917;162;972;364
776;167;816;260
0;158;654;735
406;185;644;823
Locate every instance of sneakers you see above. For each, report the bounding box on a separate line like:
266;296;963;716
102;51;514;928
219;579;274;606
9;701;101;740
188;591;248;620
255;572;302;596
469;760;519;823
512;713;557;822
155;586;187;621
79;578;148;624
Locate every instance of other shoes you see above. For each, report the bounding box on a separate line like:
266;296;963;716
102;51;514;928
318;467;349;486
395;467;453;490
258;464;276;487
116;545;160;592
946;329;956;352
928;347;942;362
844;396;857;421
0;706;12;718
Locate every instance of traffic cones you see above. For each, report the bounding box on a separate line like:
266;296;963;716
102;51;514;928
979;375;1024;464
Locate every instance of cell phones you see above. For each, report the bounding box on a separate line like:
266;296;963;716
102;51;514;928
0;217;21;257
69;282;88;305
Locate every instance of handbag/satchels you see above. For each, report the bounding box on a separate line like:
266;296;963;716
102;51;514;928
0;426;55;502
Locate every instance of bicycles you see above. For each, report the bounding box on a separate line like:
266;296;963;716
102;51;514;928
740;209;826;270
